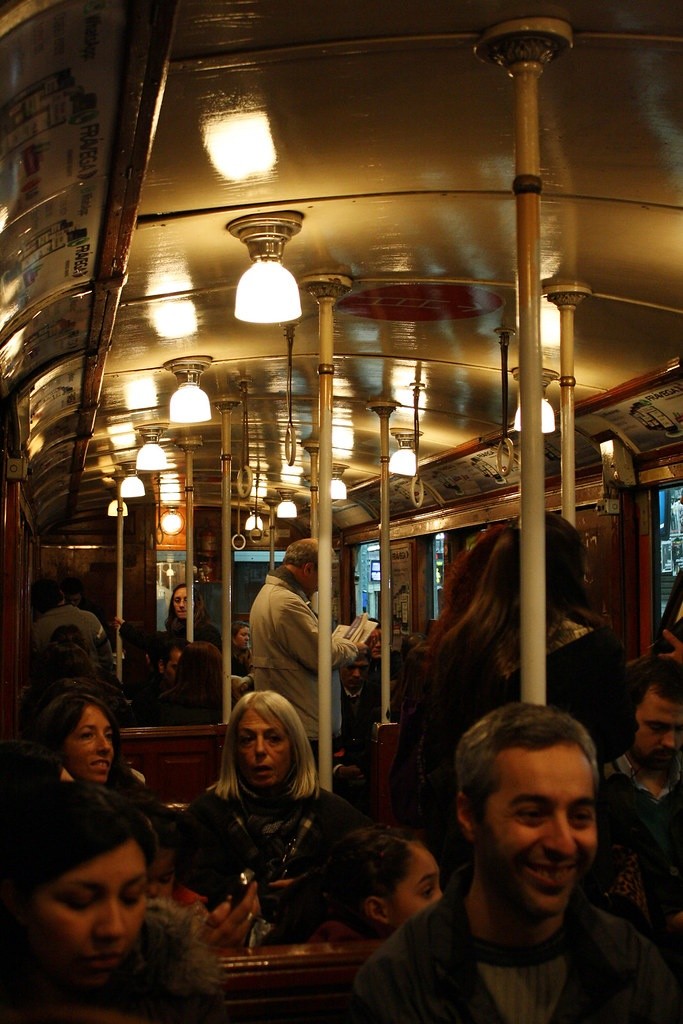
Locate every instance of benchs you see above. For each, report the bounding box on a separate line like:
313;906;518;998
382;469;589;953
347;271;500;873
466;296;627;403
118;723;404;1024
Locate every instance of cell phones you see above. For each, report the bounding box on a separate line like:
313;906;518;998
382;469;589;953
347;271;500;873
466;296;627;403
231;868;255;913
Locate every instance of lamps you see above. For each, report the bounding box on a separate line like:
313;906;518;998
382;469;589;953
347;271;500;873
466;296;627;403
134;423;169;471
274;487;299;518
162;507;181;532
107;487;128;516
244;504;265;530
163;356;212;423
512;367;560;434
330;463;349;500
388;428;424;476
120;465;146;498
227;210;303;324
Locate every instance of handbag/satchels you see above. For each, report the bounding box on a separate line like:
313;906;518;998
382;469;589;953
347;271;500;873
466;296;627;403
388;699;435;828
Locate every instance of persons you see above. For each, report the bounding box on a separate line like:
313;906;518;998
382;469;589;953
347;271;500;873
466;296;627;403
249;538;366;801
337;618;435;811
23;576;254;729
0;688;442;1023
352;703;683;1024
585;657;683;961
395;497;683;903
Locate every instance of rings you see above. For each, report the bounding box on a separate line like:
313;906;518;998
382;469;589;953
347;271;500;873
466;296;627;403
245;912;255;924
